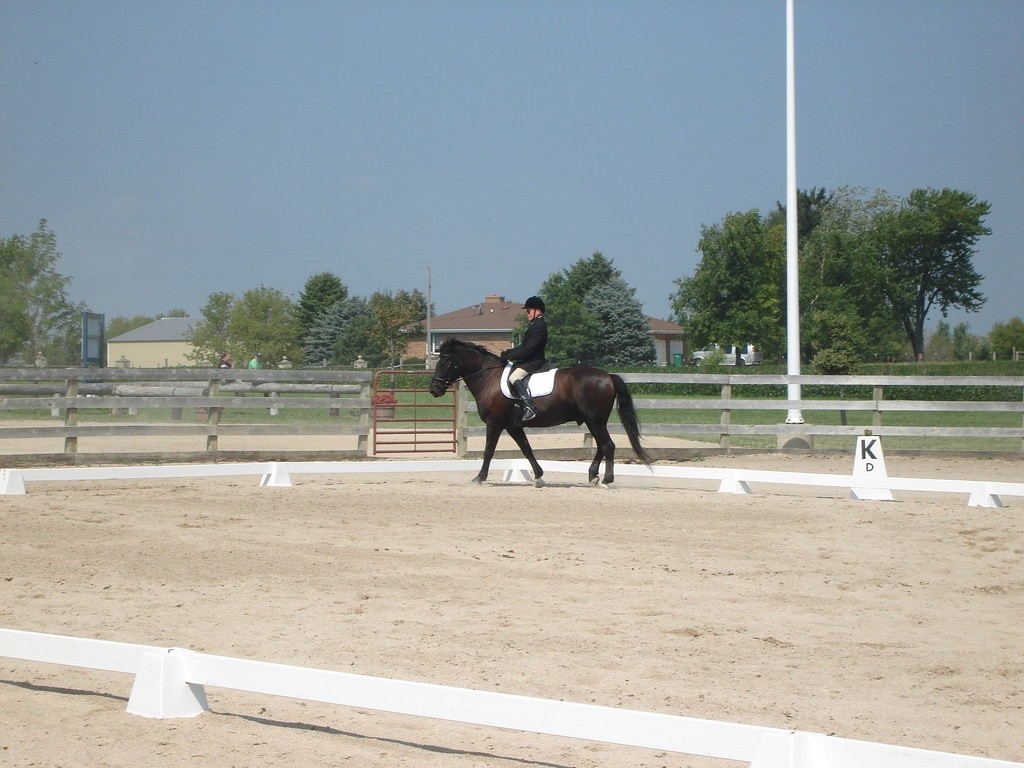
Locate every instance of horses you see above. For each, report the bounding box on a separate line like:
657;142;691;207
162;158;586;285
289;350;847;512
429;336;656;491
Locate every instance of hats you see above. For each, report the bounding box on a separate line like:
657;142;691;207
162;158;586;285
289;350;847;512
357;354;362;359
256;352;262;358
282;355;287;359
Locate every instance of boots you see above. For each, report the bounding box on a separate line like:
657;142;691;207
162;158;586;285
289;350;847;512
512;379;539;421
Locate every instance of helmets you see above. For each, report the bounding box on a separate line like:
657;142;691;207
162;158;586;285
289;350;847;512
521;296;545;313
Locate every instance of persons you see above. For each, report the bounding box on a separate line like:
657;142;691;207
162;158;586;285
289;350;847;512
500;296;548;422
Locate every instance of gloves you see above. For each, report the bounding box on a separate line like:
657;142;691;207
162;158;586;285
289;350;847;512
501;355;508;362
501;351;508;356
